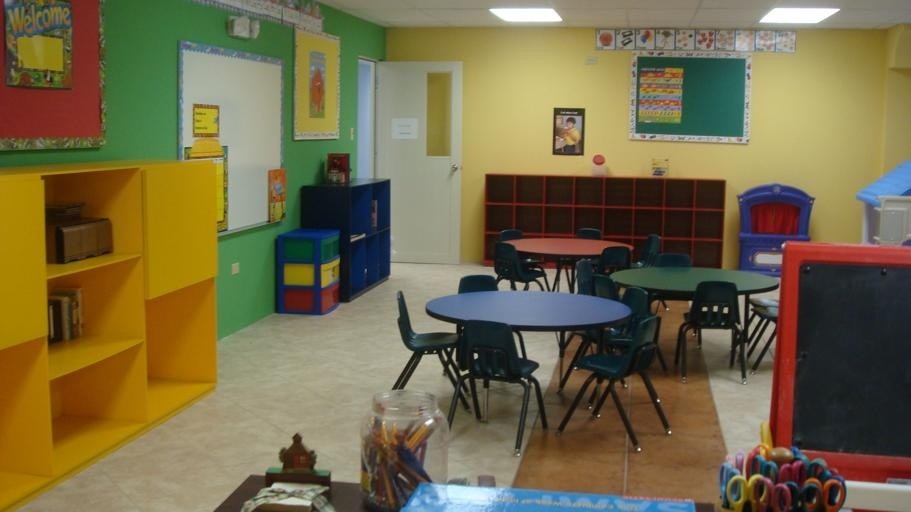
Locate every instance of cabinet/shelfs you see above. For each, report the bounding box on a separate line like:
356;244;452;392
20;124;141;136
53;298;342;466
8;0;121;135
1;154;225;510
297;176;394;304
274;226;343;317
482;172;728;270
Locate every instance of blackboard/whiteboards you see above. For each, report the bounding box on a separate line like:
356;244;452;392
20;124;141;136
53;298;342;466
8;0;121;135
629;49;753;144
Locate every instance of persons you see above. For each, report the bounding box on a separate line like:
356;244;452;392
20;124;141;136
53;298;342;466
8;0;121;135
562;117;581;152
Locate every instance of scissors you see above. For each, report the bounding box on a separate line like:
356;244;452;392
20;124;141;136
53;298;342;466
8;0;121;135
719;442;846;512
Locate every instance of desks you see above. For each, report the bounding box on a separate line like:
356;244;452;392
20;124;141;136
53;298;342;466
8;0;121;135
216;472;720;511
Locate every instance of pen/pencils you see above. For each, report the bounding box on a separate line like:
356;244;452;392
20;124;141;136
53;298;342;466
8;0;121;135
359;404;442;512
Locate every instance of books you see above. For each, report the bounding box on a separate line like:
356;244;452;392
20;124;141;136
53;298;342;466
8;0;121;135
48;285;85;345
350;233;366;242
351;234;358;239
328;152;351;182
371;199;378;228
